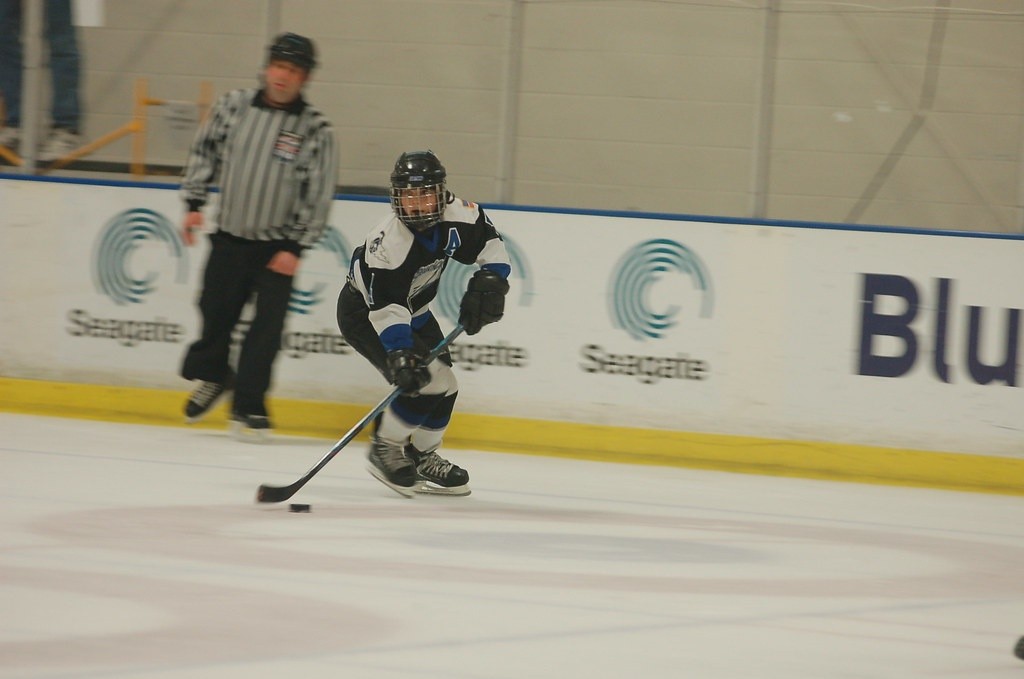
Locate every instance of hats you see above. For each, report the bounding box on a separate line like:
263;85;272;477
270;32;316;69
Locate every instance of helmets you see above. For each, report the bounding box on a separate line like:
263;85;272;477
390;149;447;229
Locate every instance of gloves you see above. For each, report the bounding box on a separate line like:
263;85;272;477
387;349;430;395
458;270;510;336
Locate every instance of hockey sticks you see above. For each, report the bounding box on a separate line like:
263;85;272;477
256;323;463;503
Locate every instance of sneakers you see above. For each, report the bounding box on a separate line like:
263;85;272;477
228;405;272;443
182;366;234;421
404;435;472;497
364;412;423;496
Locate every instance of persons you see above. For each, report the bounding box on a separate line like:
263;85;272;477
336;149;512;500
0;0;83;162
179;32;338;444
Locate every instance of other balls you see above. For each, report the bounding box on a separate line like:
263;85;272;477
287;503;311;512
